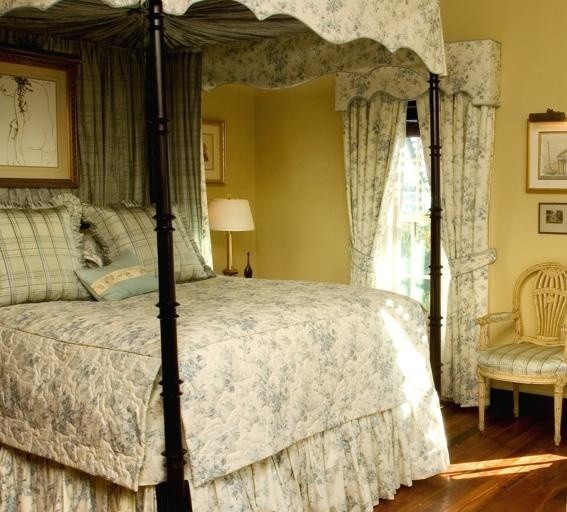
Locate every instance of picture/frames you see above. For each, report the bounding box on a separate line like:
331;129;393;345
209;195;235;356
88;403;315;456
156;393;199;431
199;116;228;189
536;202;567;235
1;42;84;194
524;113;567;196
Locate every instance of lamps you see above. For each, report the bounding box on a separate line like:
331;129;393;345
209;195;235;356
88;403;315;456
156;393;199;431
207;192;256;277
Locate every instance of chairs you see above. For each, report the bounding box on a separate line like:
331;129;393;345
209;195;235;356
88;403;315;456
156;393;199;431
470;260;567;448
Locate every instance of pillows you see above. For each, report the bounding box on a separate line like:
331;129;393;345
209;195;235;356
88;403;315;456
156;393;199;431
71;198;216;286
0;191;94;308
72;246;162;302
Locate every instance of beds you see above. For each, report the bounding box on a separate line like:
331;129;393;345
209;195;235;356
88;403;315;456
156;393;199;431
0;1;455;512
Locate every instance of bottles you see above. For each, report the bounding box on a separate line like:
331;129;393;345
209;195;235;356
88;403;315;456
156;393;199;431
244;251;253;278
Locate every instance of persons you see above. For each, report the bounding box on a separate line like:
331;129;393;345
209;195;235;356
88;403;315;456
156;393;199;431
14;77;56;167
